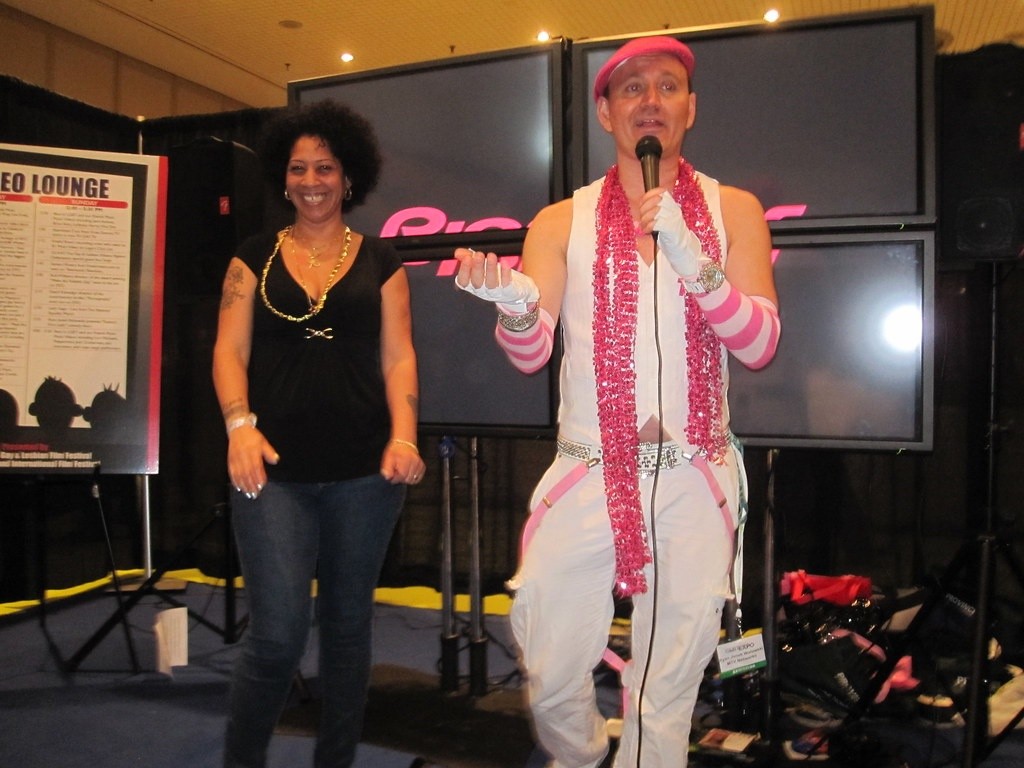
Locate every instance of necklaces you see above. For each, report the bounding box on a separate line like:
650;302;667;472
260;226;351;321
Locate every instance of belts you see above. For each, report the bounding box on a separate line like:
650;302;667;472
556;425;734;478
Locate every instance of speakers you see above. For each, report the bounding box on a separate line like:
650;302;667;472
935;42;1024;265
166;136;260;296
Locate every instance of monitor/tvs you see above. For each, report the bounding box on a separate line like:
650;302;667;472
285;5;935;452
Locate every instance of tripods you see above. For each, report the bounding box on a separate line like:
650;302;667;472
58;484;248;673
839;265;1023;768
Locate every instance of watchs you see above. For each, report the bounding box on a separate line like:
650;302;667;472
682;260;726;293
226;413;257;432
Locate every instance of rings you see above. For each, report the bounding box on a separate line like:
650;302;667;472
414;474;418;479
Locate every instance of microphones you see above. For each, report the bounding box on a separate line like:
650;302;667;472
635;136;663;241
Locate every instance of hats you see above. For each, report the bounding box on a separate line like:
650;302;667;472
594;36;694;104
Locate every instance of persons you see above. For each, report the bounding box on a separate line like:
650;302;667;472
454;37;781;768
212;105;425;767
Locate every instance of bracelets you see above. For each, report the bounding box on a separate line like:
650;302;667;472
497;299;540;331
387;440;419;453
